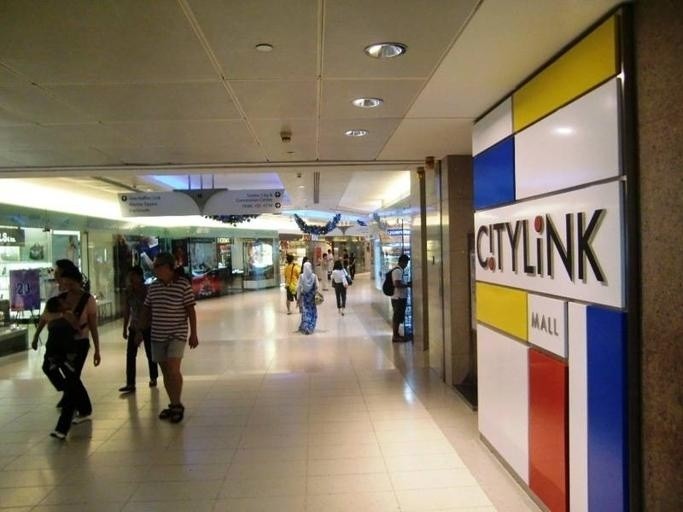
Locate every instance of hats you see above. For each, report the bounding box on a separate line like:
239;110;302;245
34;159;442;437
398;255;410;261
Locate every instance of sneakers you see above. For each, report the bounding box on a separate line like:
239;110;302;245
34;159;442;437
48;430;67;439
71;415;91;424
118;385;135;392
392;335;409;342
149;377;156;386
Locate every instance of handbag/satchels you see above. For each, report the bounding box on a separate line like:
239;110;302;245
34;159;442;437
314;289;324;305
382;271;393;296
342;273;352;289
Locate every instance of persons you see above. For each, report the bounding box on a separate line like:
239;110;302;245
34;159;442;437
32;259;78;408
134;253;199;425
31;267;100;440
112;234;132;294
137;235;161;282
283;249;356;317
390;254;412;344
295;261;318;336
118;266;158;394
65;235;77;262
248;241;264;270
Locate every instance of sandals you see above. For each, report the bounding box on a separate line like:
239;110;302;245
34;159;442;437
158;402;184;423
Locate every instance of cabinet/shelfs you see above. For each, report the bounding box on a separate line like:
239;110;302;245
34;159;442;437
386;217;413;339
